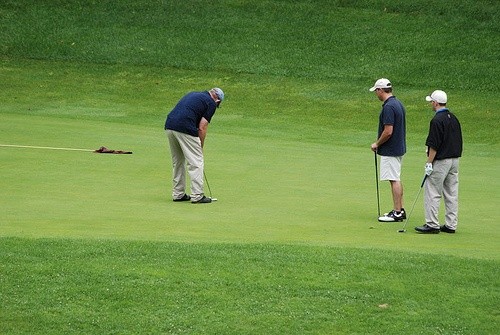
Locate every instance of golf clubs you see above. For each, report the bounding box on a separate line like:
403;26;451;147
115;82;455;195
399;174;429;232
374;149;380;216
203;169;217;202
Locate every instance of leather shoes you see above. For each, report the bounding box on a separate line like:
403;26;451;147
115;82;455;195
440;225;455;233
173;194;191;201
414;223;441;234
192;196;211;203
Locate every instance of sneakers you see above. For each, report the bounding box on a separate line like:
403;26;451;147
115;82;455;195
378;208;406;222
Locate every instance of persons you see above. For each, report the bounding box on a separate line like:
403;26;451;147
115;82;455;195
369;78;406;223
164;88;224;203
414;90;463;234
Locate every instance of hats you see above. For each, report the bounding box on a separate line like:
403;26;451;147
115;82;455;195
369;78;392;92
212;88;224;108
425;90;447;103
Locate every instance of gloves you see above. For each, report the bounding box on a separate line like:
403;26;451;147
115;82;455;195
424;162;433;176
